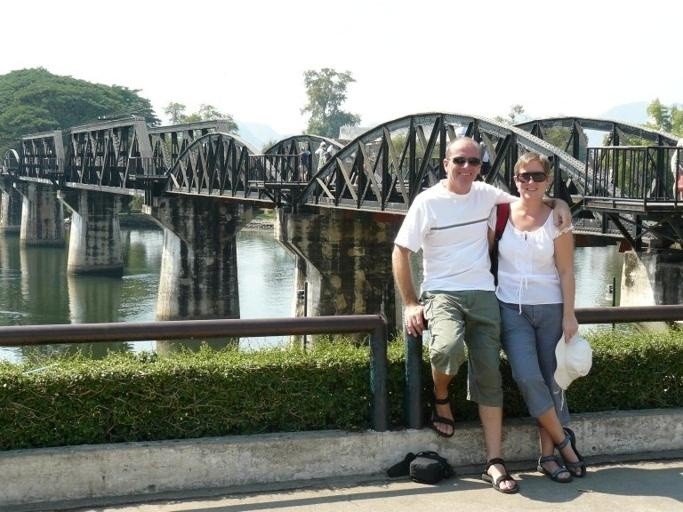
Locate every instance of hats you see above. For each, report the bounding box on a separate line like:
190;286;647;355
552;326;594;392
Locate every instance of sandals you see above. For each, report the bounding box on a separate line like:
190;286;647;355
431;393;456;436
481;458;519;495
554;428;586;478
535;453;573;485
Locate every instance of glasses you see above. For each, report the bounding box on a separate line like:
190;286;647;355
515;172;547;184
444;154;483;167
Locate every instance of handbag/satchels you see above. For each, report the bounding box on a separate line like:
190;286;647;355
407;449;455;485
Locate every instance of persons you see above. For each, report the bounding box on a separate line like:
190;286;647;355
388;134;571;495
487;149;587;483
314;137;334;184
297;147;311;183
670;137;683;201
477;135;492;181
